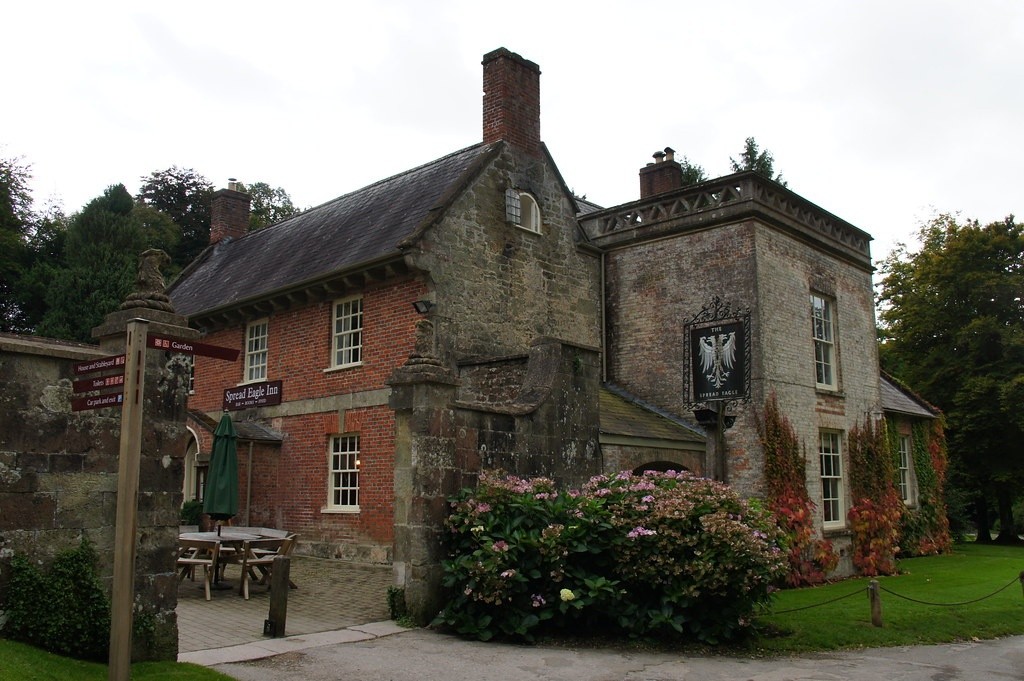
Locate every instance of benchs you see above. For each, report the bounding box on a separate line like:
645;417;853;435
178;533;299;601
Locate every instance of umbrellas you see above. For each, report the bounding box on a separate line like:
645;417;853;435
202;407;244;585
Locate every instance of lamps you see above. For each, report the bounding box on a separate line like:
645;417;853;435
413;299;433;314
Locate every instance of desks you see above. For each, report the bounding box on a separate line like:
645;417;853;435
179;532;262;590
